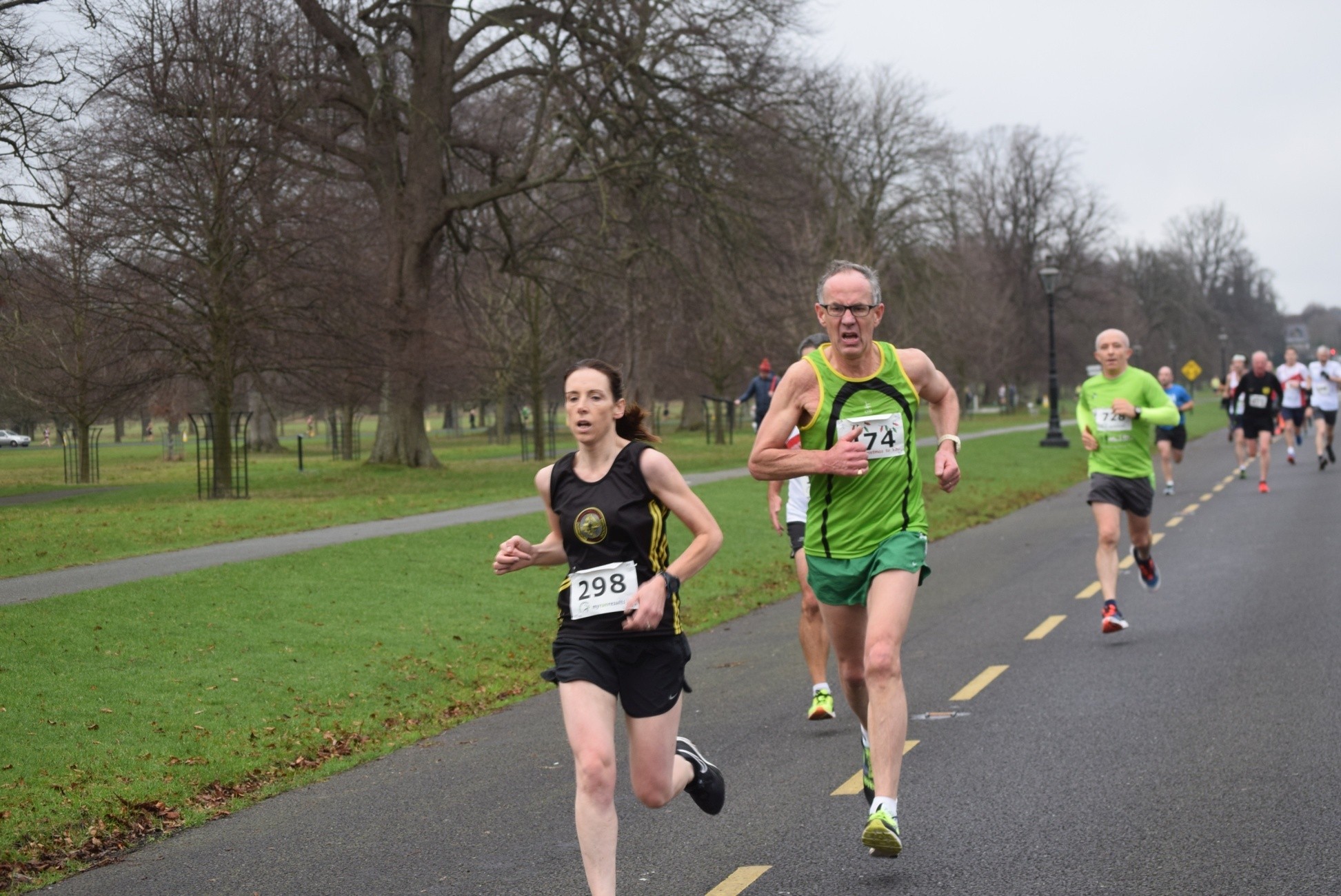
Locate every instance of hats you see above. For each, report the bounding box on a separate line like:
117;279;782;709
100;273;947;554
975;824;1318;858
760;359;771;371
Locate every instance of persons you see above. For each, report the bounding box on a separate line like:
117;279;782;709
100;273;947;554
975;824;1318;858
733;358;781;432
768;334;838;720
1155;366;1193;495
1305;345;1341;470
747;259;960;858
1214;347;1313;493
1075;329;1181;632
493;359;725;896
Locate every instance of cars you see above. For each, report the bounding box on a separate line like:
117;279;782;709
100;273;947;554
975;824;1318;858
0;429;31;447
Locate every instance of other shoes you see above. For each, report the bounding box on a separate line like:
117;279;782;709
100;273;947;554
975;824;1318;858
1241;470;1246;479
1287;456;1295;465
1326;445;1335;463
1163;486;1173;495
1318;457;1326;470
1295;435;1302;444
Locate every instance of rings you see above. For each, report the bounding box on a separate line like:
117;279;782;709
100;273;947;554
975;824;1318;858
858;469;862;476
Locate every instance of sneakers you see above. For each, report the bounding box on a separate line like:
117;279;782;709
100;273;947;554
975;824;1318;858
676;737;725;815
861;738;874;806
1102;604;1129;632
1260;482;1269;492
1130;545;1162;593
862;810;902;858
808;689;835;719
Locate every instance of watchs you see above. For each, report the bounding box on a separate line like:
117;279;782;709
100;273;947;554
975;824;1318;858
1134;406;1141;419
937;434;961;452
658;570;680;593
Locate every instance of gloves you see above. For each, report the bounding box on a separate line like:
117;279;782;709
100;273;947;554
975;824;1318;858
1320;371;1329;380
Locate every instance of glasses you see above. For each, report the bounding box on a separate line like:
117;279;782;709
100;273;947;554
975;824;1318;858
819;304;878;316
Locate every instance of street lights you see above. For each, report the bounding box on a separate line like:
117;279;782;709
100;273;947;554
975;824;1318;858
1219;334;1228;409
1037;254;1070;447
